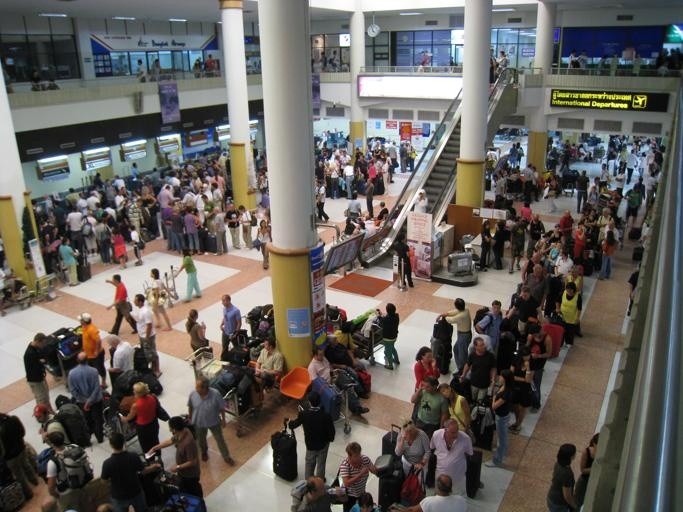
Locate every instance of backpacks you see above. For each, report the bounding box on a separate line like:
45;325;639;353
474;306;494;334
42;403;92;448
48;443;94;492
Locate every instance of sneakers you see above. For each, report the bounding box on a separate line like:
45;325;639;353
485;459;496;467
202;453;208;461
224;457;234;466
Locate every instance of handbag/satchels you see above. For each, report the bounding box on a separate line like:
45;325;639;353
148;293;165;306
328;487;349;504
158;406;169;421
0;481;25;511
400;464;426;505
434;318;452;340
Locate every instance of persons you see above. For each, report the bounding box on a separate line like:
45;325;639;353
2;47;683;511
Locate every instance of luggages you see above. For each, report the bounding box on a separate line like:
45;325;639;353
383;423;402;454
230;332;249;365
378;473;401;512
431;338;452;374
542;311;563;357
163;484;206;511
271;417;297;481
470;398;493;450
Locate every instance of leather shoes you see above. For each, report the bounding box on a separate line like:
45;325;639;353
353;406;369;413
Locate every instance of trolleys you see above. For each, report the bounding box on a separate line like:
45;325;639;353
351;321;385;365
185;346;263;437
141;265;179;308
40;327;82;388
297;366;360;434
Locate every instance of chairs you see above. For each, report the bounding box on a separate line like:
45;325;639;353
279;367;313;401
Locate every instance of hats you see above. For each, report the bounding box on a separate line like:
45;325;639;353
46;420;64;434
32;404;49;417
77;312;91;323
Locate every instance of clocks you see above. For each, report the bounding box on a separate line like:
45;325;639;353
367;12;381;39
